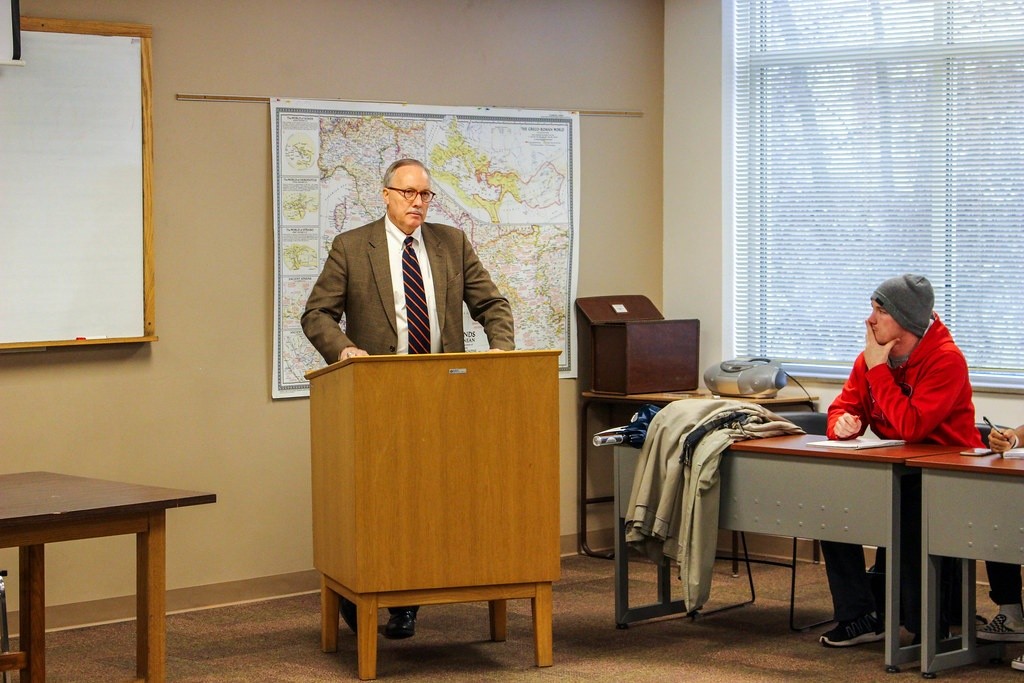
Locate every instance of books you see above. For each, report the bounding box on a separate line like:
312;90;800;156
1003;448;1024;459
807;437;907;450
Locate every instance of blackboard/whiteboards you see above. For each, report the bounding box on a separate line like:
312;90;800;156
0;16;160;353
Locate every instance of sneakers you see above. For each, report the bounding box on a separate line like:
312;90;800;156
816;614;886;647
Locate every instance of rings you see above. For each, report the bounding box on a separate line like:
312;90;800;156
347;353;351;355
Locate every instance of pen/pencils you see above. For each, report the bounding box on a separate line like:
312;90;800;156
983;416;1012;445
855;416;859;422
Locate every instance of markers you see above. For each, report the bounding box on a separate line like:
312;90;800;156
76;336;107;340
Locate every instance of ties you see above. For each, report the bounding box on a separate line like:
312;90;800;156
402;236;431;354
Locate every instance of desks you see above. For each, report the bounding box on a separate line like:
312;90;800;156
613;434;971;674
0;470;216;683
905;447;1024;678
576;386;822;579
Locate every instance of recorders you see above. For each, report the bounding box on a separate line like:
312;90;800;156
704;359;787;398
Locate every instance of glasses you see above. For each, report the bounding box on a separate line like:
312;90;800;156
387;187;436;203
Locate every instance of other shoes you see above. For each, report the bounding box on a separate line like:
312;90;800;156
974;614;1024;642
1011;654;1024;671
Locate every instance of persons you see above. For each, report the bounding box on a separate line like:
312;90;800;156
818;273;986;647
974;423;1024;670
299;159;515;638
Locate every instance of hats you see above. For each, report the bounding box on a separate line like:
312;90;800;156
869;272;935;338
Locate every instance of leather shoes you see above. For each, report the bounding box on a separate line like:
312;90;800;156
383;609;416;637
340;596;358;631
